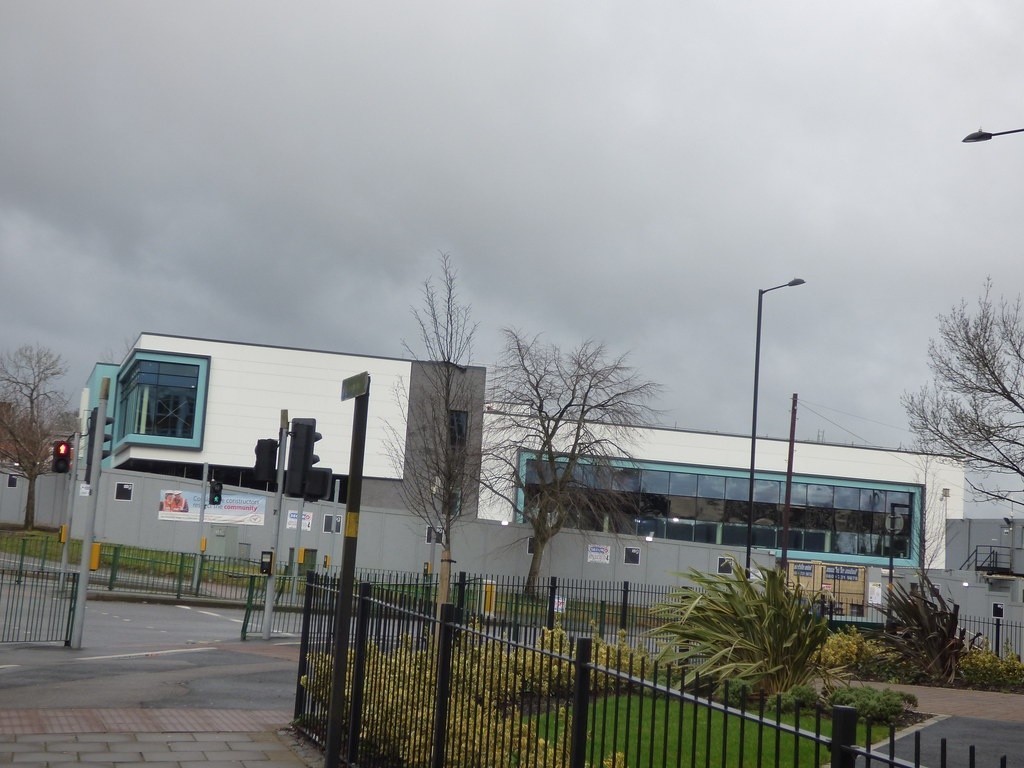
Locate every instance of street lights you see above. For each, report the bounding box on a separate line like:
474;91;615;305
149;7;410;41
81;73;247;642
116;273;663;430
942;487;950;570
326;369;372;768
745;276;806;580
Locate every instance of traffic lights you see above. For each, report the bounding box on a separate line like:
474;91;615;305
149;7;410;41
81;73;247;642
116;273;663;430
282;417;323;498
52;439;71;474
208;482;221;505
